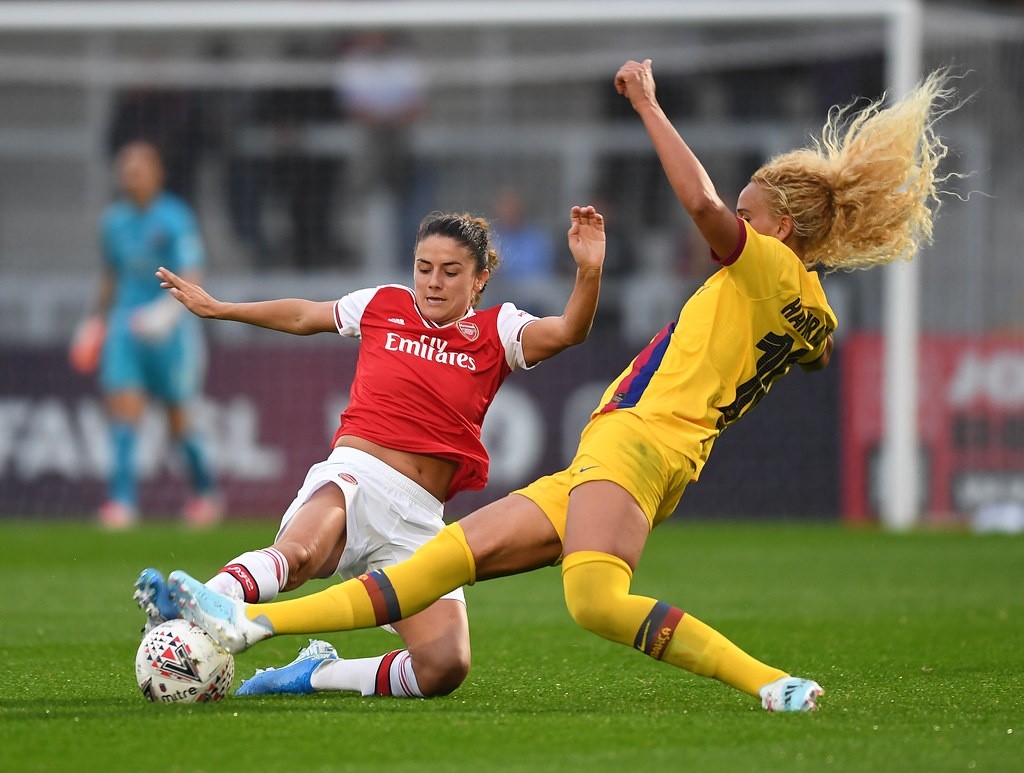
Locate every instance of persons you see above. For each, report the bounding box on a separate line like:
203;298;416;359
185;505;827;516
167;57;955;711
135;205;606;698
69;138;219;526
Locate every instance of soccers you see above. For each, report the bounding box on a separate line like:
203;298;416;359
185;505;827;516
134;618;234;703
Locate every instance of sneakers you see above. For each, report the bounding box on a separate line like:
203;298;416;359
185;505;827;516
181;495;228;531
168;569;272;653
235;640;341;698
758;677;825;715
97;501;139;531
133;569;177;639
